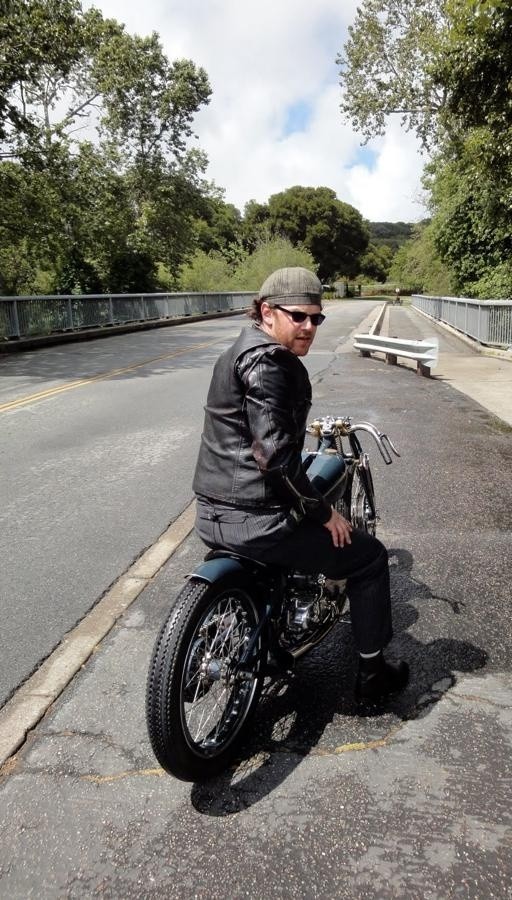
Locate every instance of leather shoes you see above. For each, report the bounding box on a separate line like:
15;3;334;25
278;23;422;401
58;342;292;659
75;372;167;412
348;660;410;699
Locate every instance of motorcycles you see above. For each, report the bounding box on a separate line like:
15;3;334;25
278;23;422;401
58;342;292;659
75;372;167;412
144;413;399;784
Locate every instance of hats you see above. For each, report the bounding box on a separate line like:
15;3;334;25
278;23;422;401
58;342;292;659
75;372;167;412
259;267;324;309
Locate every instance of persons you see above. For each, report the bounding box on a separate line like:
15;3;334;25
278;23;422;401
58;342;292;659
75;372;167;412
192;267;411;700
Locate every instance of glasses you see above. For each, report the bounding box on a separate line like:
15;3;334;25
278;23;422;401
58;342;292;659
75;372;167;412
274;305;326;326
